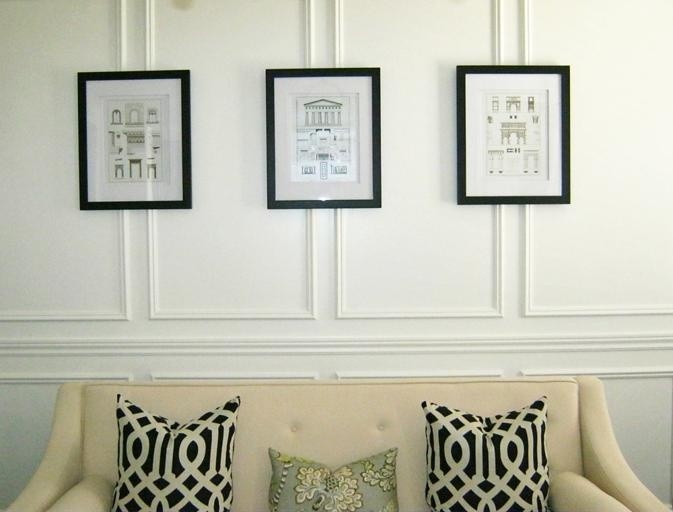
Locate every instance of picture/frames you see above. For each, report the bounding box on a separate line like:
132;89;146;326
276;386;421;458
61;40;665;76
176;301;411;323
453;65;570;208
262;66;386;210
77;67;193;209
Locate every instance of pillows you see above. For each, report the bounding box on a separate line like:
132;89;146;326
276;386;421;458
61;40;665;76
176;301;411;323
418;393;551;510
117;395;244;510
265;446;403;510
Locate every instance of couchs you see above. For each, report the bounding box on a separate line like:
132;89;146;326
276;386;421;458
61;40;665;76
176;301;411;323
3;377;671;512
